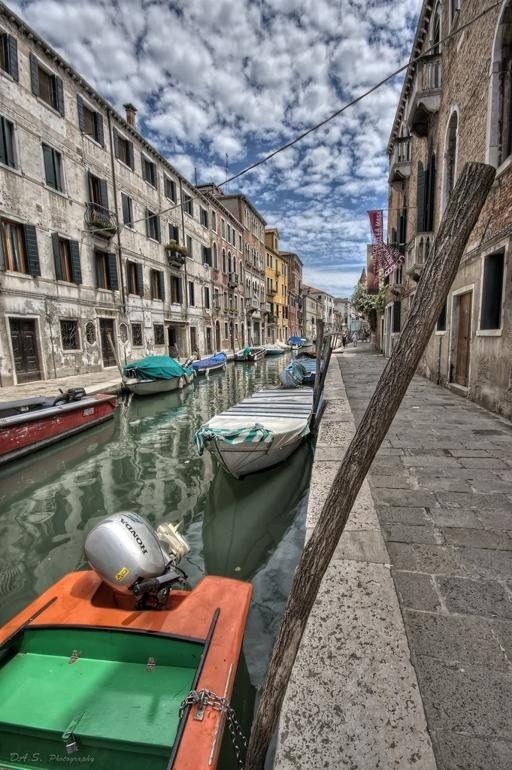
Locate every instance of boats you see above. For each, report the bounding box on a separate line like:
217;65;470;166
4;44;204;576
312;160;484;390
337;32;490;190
122;355;196;394
0;505;262;770
0;385;116;463
191;340;320;478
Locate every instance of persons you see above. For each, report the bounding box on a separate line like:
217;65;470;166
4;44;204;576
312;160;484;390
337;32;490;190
351;332;359;347
342;332;347;347
